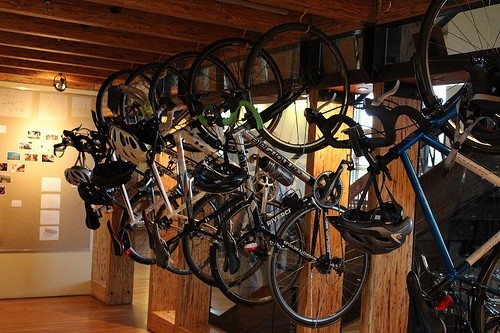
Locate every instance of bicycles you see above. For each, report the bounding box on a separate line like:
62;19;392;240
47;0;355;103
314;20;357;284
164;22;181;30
53;0;500;333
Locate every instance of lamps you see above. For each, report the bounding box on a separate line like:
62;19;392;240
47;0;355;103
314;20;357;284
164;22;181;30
52;73;67;91
